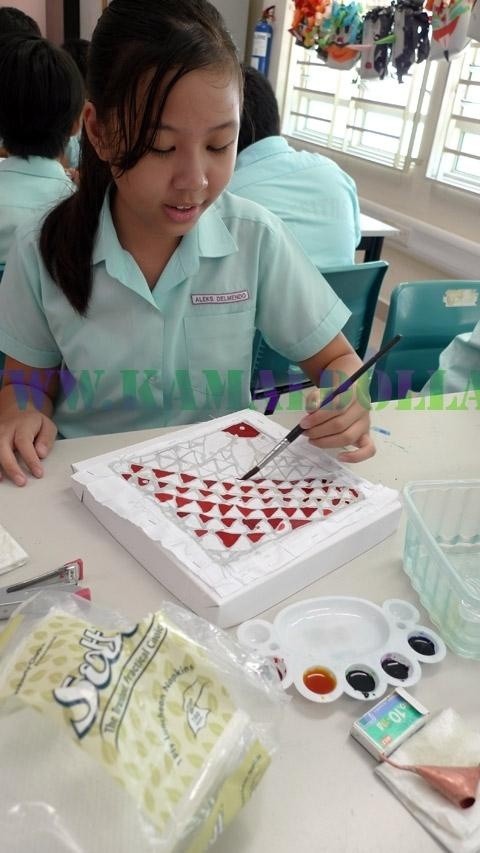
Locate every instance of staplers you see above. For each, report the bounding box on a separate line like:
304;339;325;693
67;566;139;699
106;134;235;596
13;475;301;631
0;559;91;621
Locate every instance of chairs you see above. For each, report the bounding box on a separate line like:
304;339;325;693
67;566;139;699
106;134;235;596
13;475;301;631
253;261;480;403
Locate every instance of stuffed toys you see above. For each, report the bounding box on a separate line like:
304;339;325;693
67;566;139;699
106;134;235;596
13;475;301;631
289;0;480;84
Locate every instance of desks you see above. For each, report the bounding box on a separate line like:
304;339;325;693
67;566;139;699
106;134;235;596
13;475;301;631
0;390;480;853
357;213;401;265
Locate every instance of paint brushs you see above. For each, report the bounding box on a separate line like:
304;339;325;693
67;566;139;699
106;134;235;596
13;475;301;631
240;334;402;481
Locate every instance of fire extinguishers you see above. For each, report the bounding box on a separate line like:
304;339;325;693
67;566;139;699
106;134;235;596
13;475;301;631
248;5;275;80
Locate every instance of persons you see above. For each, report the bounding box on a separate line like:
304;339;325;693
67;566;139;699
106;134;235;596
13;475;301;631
0;0;378;486
224;60;365;271
62;37;95;78
1;36;89;266
2;8;42;42
